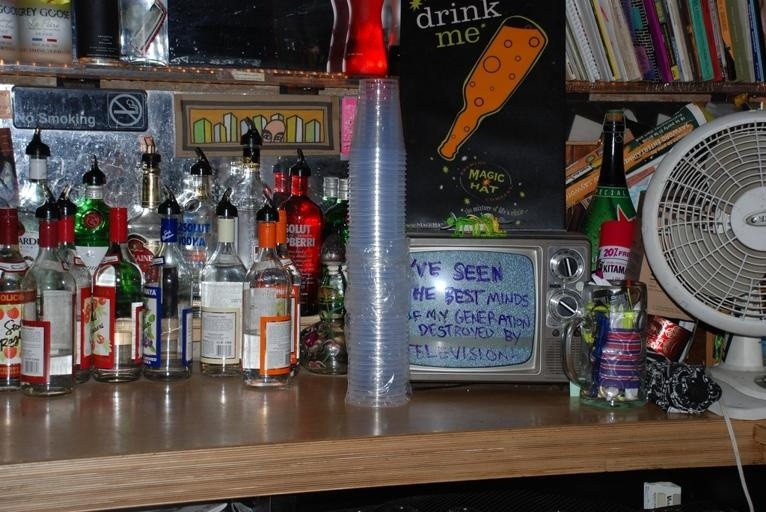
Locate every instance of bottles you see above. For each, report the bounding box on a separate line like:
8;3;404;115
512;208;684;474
587;111;637;293
0;116;348;396
437;13;548;160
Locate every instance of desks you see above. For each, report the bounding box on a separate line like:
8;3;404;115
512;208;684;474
0;345;766;512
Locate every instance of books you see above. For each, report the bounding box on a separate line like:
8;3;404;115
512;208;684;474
566;2;765;80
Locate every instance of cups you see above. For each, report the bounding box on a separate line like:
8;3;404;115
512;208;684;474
560;283;648;410
347;77;407;407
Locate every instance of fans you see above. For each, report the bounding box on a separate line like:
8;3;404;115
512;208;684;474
642;111;766;421
